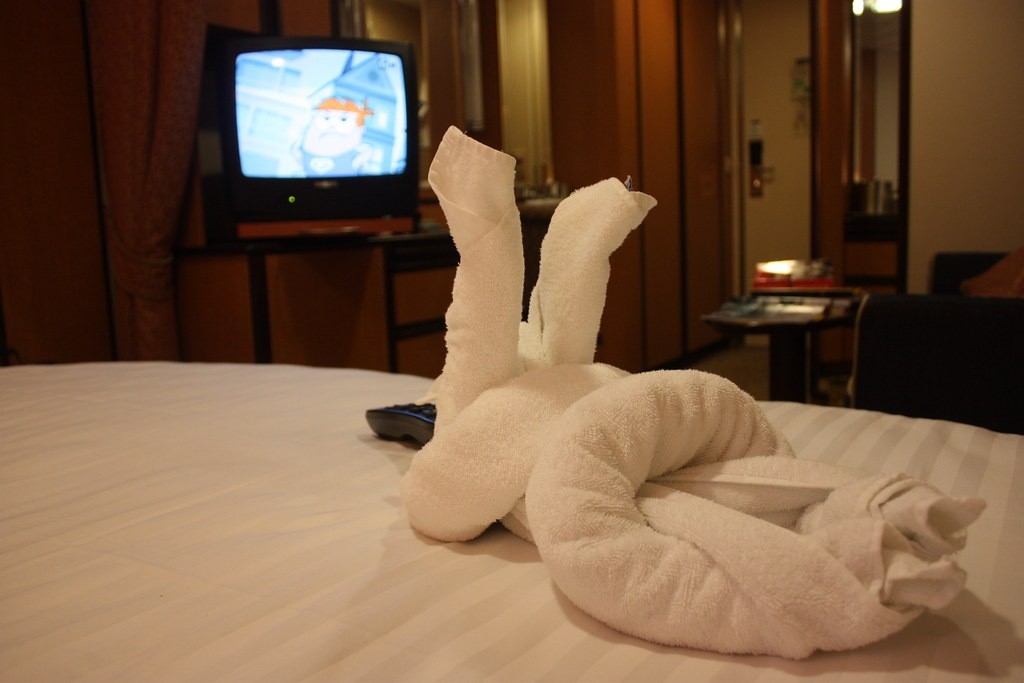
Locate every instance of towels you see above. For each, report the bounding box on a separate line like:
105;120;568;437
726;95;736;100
404;124;989;663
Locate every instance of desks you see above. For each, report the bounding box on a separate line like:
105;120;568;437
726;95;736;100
709;316;854;403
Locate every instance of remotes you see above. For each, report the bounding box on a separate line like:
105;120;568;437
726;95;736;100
364;402;438;445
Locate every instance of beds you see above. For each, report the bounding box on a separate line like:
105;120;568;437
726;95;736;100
0;362;1024;683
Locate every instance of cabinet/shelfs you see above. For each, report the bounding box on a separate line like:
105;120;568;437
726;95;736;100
175;214;458;377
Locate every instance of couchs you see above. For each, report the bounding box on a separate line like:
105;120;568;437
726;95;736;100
863;253;1024;434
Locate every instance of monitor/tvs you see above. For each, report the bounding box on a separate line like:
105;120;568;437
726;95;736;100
215;34;420;243
